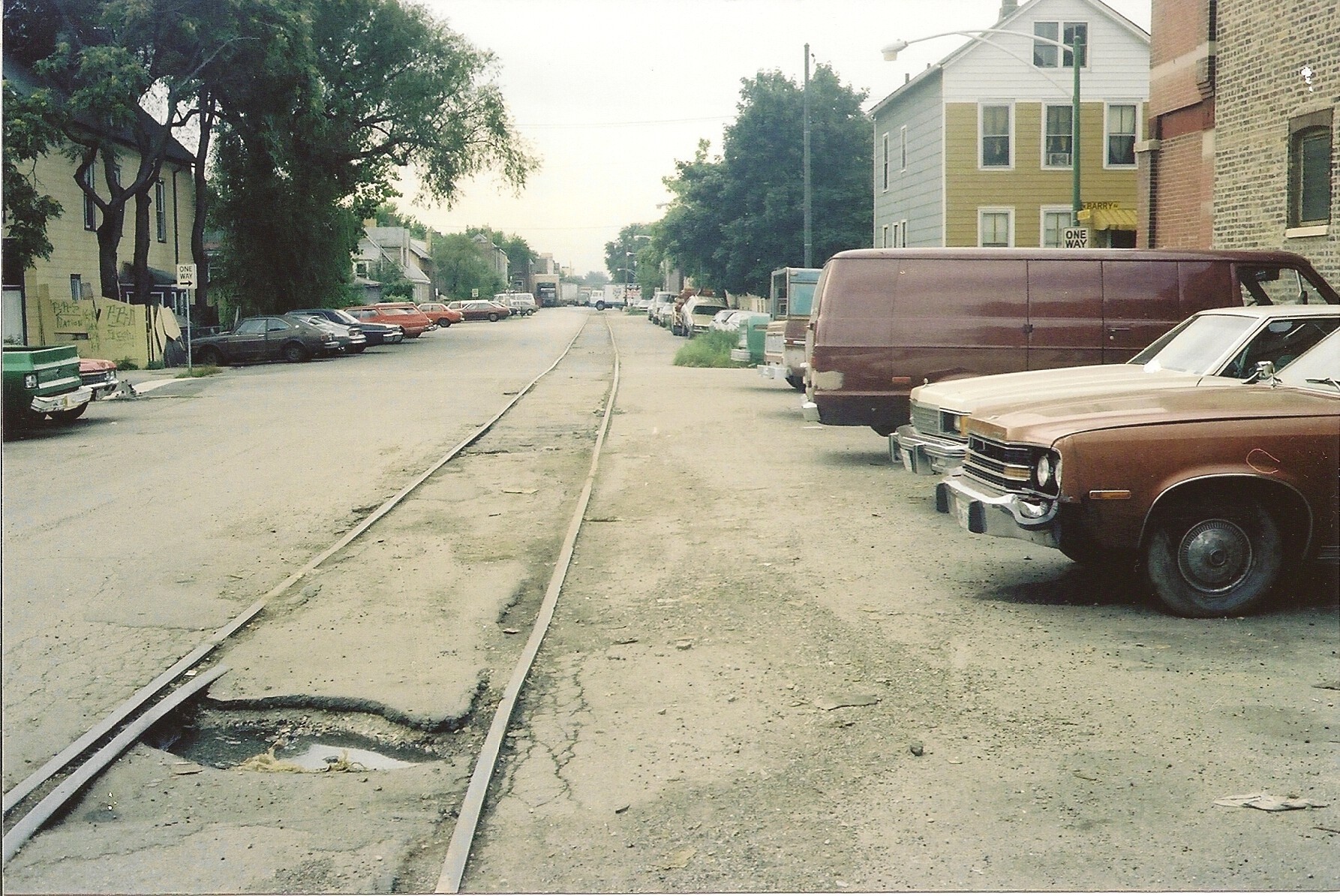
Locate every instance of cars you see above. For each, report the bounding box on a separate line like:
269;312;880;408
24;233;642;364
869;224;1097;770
933;326;1340;619
887;304;1340;476
47;358;120;420
417;299;539;328
636;289;770;358
191;301;438;367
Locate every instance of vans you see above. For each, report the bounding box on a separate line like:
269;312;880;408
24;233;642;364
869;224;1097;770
799;247;1340;438
493;293;536;305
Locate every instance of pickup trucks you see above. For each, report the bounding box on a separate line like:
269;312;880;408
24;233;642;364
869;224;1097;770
730;315;770;363
3;343;91;422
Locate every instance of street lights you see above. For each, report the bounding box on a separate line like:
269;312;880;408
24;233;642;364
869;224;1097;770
880;28;1081;248
656;202;704;288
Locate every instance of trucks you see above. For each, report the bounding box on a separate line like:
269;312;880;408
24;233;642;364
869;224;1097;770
589;283;642;311
756;266;822;393
536;282;557;308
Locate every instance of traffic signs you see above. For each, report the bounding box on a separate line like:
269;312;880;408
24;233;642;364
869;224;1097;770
1061;226;1091;249
176;263;197;290
